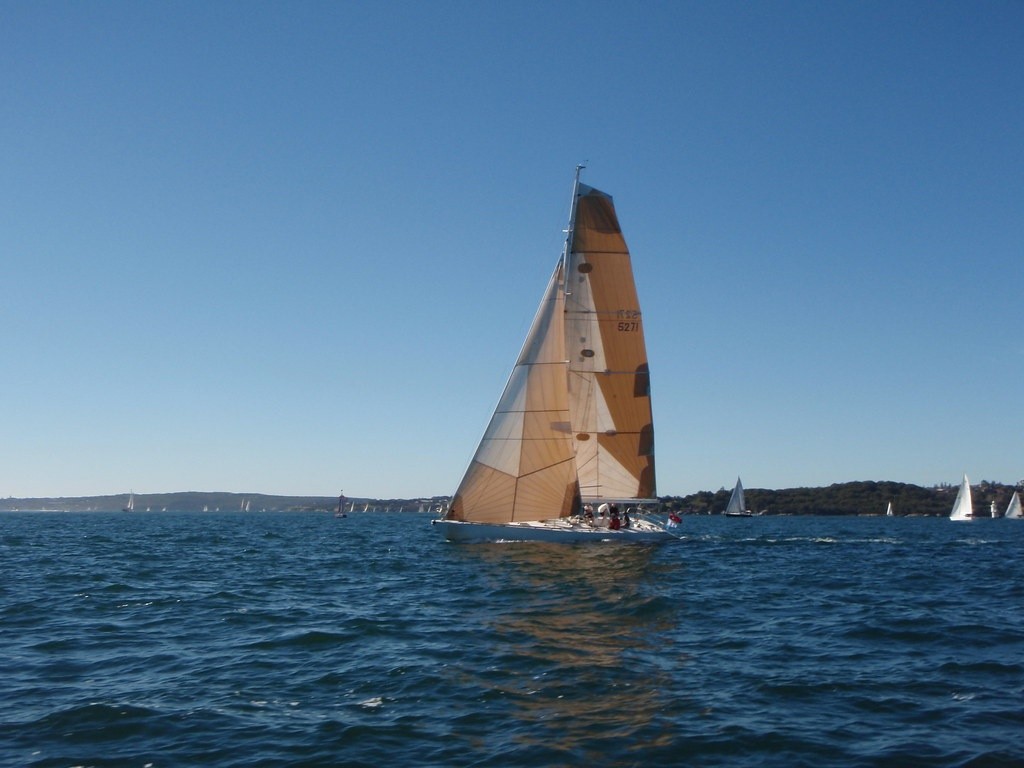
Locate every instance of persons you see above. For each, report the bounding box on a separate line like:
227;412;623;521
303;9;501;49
584;503;595;527
598;502;630;530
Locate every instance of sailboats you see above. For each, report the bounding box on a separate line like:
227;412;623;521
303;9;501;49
122;486;137;513
723;475;753;518
335;489;350;519
429;162;686;546
1002;491;1024;520
949;473;978;522
